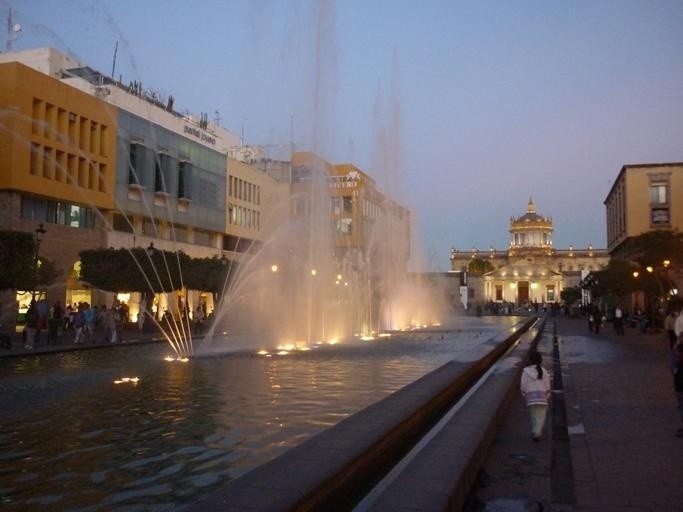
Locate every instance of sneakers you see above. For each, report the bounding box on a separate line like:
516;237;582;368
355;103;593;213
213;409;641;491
532;433;544;441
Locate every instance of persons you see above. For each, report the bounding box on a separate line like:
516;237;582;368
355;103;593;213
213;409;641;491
664;297;683;399
613;300;625;336
22;300;207;350
520;351;551;442
460;298;608;336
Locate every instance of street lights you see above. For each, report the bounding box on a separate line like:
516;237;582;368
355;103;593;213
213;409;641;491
219;255;228;291
305;267;318;343
27;224;46;306
143;242;158;258
633;260;678;336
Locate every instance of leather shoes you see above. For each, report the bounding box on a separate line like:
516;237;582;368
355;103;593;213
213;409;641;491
674;428;683;439
